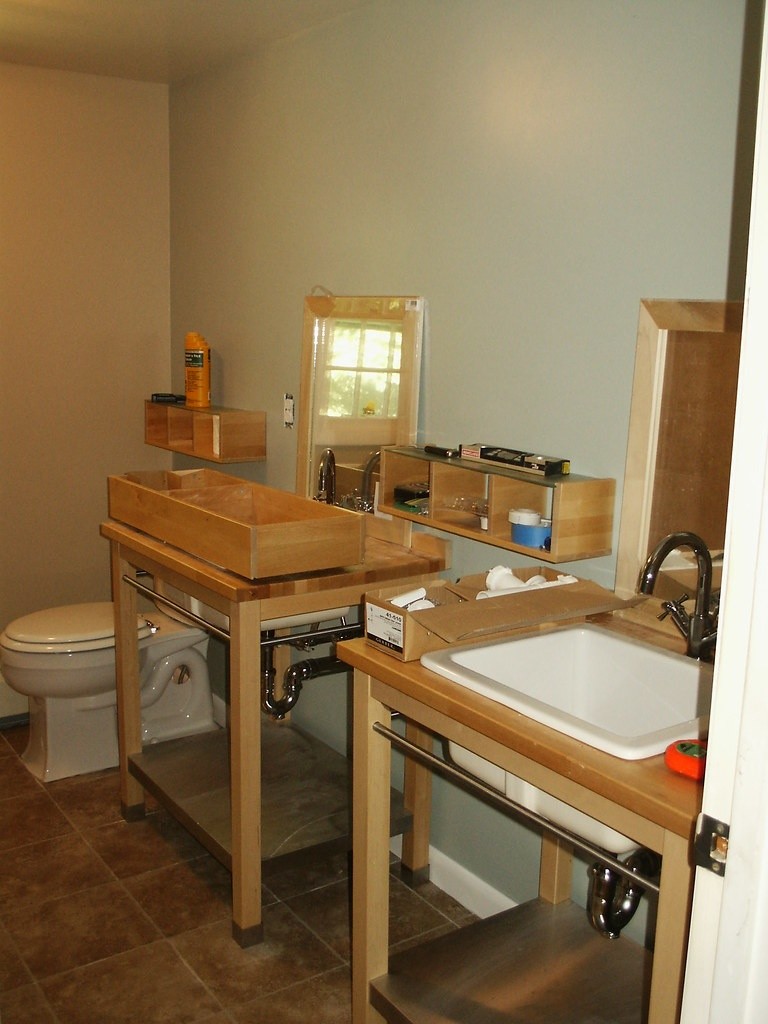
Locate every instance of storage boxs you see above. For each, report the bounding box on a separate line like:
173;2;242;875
460;443;571;477
364;566;649;663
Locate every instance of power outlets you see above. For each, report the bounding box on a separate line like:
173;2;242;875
284;399;294;423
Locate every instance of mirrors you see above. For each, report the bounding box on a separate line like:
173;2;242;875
613;298;743;640
295;296;426;548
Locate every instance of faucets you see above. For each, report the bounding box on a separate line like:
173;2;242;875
637;531;712;651
359;452;381;496
317;448;337;504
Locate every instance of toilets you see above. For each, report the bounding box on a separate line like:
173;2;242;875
0;603;210;783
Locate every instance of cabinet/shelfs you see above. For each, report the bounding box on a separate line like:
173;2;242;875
335;465;381;515
336;613;717;1024
377;446;616;564
108;468;367;580
144;398;266;464
99;522;452;929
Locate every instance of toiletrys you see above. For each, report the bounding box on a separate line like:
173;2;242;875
183;329;211;408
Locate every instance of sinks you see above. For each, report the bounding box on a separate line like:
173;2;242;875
419;625;715;860
107;466;251;541
158;484;366;580
